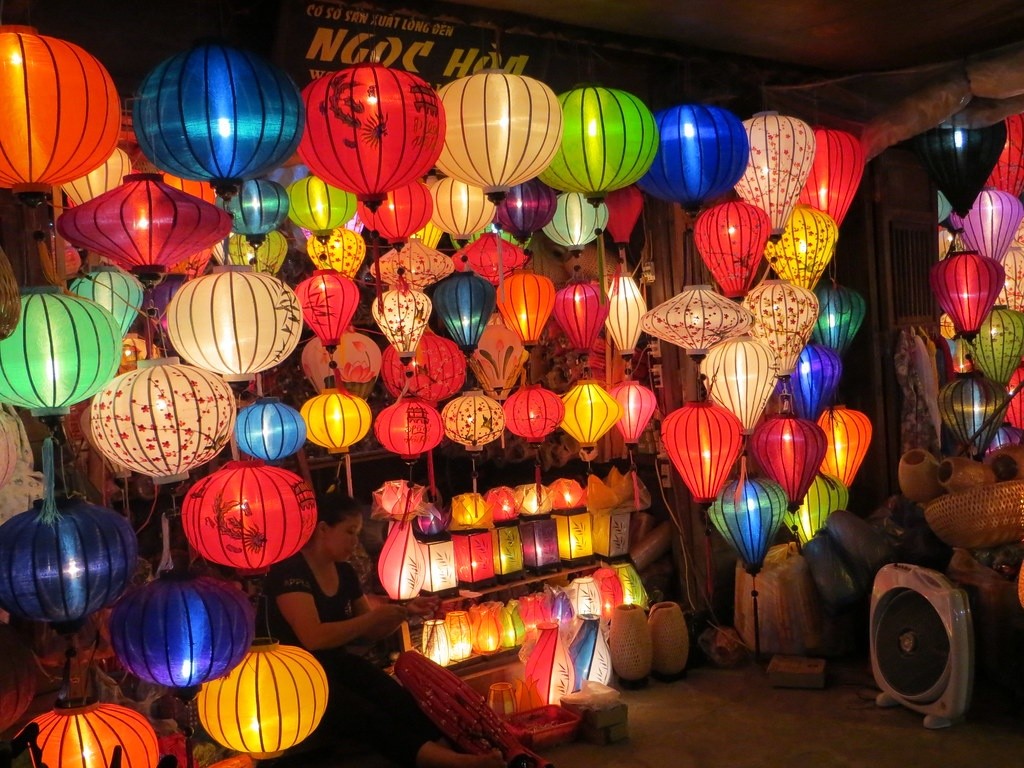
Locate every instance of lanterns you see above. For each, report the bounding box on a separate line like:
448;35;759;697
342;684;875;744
0;25;873;768
913;113;1024;452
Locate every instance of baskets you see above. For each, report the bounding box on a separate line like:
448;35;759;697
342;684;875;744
506;705;580;750
925;480;1024;549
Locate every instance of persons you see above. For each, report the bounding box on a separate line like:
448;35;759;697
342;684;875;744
254;495;503;768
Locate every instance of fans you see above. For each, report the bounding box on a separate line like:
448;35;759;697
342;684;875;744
869;562;972;728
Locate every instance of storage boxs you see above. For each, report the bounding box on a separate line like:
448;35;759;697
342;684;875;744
504;704;581;748
